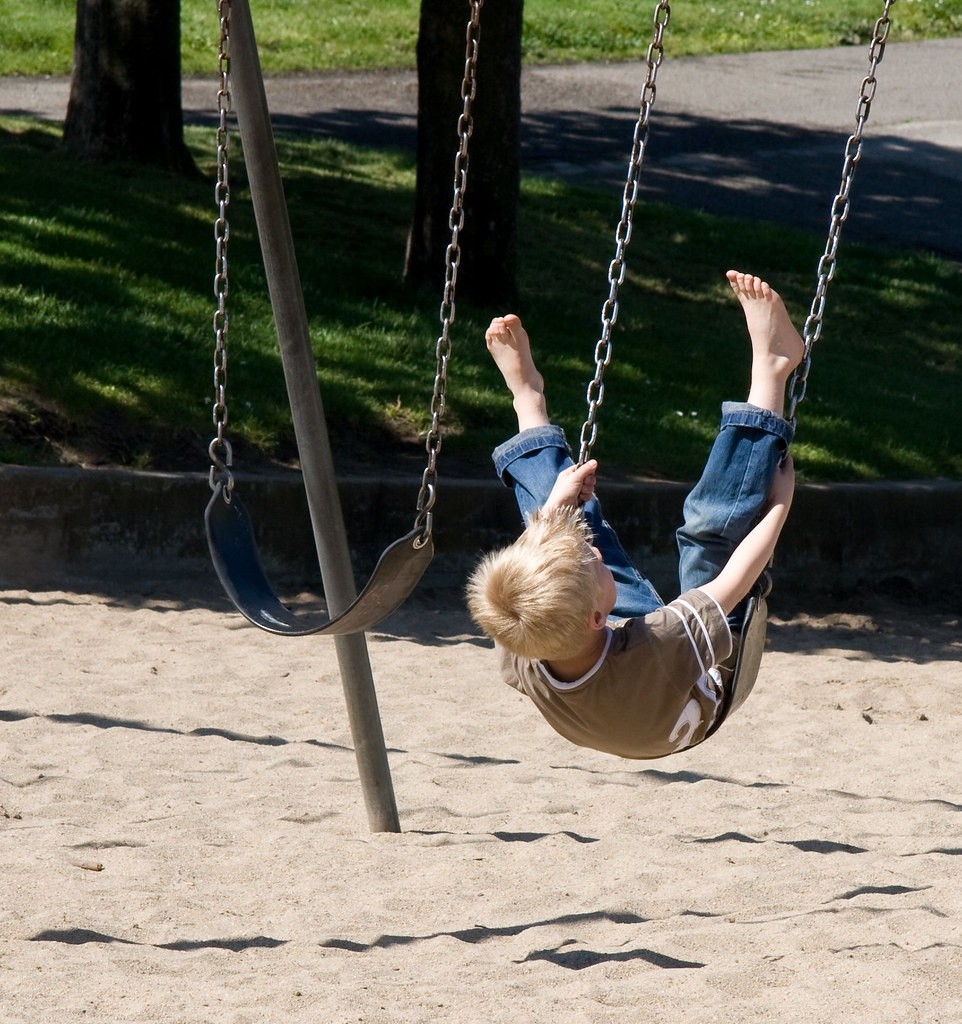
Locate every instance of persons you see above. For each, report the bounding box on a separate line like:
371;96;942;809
470;271;804;759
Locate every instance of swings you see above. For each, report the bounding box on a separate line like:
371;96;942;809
198;0;488;638
570;1;896;738
